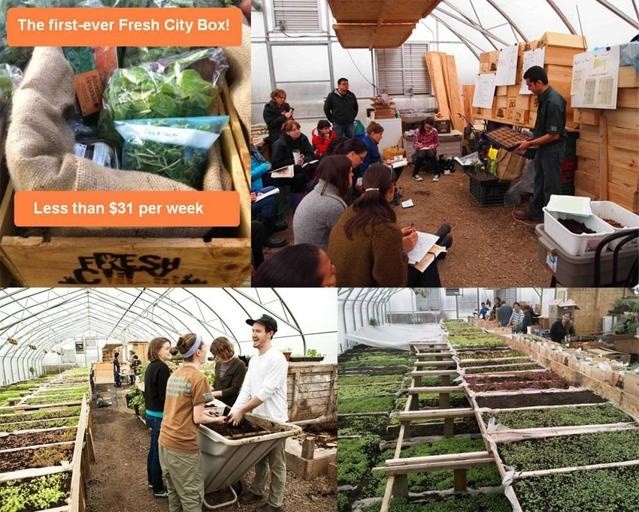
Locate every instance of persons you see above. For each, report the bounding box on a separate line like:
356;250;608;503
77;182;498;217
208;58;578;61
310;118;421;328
251;65;567;287
113;314;289;511
478;298;575;344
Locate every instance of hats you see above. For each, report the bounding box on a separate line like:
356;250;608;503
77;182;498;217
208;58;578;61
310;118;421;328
246;313;276;330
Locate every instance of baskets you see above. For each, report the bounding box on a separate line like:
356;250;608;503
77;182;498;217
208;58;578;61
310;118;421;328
465;170;510;207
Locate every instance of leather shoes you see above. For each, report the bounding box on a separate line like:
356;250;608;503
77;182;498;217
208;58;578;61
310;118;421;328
261;236;288;248
272;219;289;233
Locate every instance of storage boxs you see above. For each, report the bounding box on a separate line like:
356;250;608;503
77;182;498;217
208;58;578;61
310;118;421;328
469;31;639;287
435;120;452;133
0;79;251;288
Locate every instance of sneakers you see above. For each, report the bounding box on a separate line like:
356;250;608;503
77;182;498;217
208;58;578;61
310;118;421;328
437;235;453;260
239;492;266;503
148;484;168;497
435;224;450;245
432;175;440;182
255;503;285;512
513;209;541;220
411;174;424;181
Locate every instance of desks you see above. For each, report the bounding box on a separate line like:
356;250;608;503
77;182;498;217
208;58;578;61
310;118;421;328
404;129;466;162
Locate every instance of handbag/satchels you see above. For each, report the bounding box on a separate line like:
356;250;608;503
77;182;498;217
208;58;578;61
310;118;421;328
495;146;526;183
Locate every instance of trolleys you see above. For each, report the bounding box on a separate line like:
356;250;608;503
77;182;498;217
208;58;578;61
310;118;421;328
536;216;639;287
195;407;302;511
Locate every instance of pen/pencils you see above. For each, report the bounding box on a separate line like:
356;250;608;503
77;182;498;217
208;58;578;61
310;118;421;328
410;222;415;232
